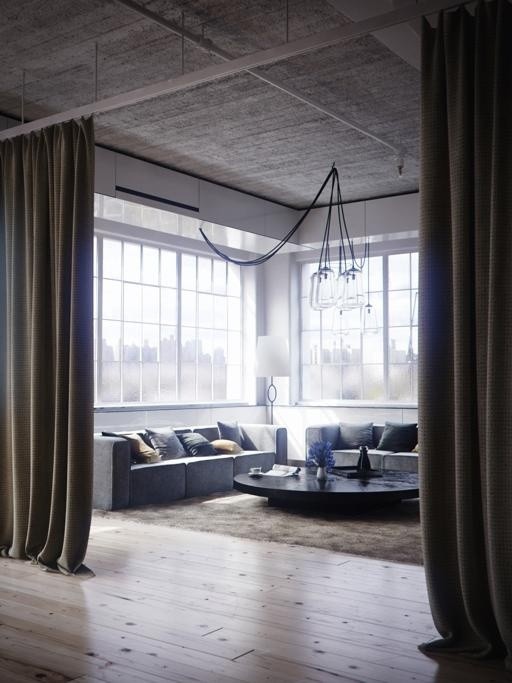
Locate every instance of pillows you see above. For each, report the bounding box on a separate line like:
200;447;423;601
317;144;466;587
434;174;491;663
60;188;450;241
102;432;139;464
125;433;160;463
211;439;242;454
217;421;242;448
376;422;418;452
176;432;217;457
339;421;374;450
144;427;188;461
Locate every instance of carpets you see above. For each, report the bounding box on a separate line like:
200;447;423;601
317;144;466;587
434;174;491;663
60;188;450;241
91;488;424;567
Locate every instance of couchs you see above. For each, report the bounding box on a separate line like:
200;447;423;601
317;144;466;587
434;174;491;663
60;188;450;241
305;425;418;474
91;424;288;511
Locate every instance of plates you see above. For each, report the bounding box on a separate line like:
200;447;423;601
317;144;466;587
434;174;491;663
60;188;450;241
248;473;263;476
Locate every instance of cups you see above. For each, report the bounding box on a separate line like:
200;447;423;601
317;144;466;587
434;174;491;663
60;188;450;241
251;468;261;474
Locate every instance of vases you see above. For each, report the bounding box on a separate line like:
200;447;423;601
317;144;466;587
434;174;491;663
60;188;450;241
317;467;328;480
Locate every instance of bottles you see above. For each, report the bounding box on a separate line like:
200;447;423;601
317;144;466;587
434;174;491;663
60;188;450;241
357;444;371;474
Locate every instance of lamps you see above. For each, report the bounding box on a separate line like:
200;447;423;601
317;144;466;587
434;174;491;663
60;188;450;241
254;335;290;425
199;166;378;335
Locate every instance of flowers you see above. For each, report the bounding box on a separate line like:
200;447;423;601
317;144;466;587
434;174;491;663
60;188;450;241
305;441;335;468
